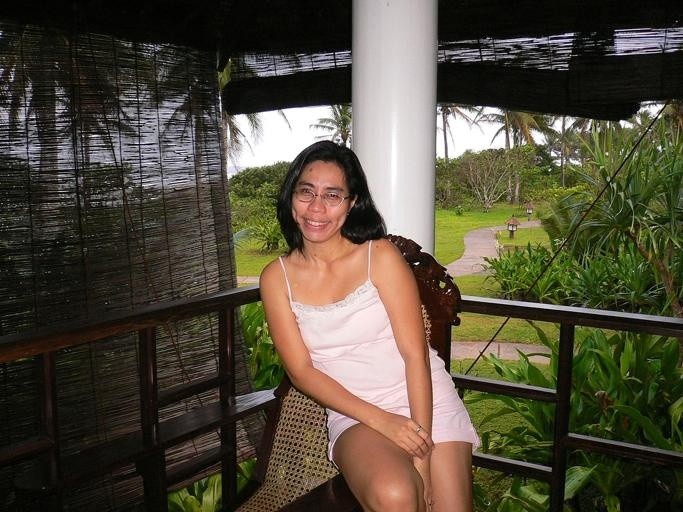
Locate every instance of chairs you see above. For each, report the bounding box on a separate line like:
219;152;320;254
217;234;462;512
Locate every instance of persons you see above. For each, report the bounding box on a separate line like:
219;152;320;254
259;140;483;512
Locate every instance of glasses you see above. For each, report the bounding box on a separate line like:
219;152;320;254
296;189;349;207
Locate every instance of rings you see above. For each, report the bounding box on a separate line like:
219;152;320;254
416;426;423;432
